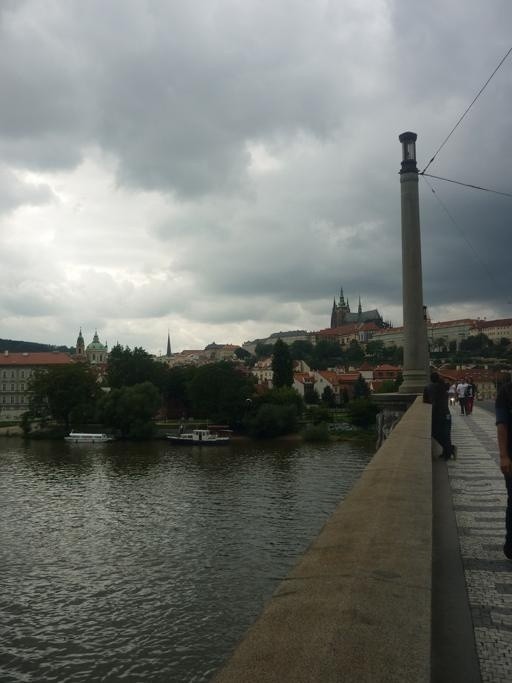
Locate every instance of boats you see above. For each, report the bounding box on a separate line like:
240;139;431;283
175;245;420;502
165;427;230;446
64;428;114;443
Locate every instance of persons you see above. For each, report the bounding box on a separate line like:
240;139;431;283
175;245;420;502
427;372;457;461
449;378;475;415
495;381;512;559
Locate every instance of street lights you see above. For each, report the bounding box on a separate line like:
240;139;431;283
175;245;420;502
394;129;432;393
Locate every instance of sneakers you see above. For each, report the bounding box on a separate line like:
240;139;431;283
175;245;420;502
439;445;457;461
